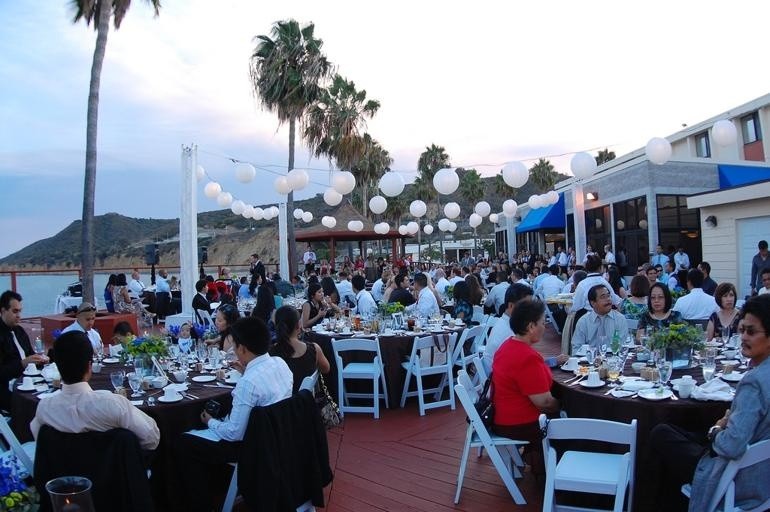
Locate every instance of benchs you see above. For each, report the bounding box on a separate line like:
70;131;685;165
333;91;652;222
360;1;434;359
37;309;137;346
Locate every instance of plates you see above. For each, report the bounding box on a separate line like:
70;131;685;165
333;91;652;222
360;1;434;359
15;335;245;408
555;322;754;402
316;305;470;339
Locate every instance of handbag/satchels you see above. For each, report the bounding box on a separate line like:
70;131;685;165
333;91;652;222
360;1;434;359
315;395;345;431
465;399;495;430
419;330;452;367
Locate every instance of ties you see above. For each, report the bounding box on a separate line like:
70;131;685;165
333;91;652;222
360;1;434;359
597;315;607;346
11;331;26;360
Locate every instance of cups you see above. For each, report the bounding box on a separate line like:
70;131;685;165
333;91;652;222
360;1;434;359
44;475;95;512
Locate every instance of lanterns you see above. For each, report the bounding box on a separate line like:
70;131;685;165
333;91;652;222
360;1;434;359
712;120;737;147
196;150;596;237
646;136;673;165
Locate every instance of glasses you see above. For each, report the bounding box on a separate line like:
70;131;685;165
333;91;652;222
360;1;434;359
78;306;96;314
739;326;765;335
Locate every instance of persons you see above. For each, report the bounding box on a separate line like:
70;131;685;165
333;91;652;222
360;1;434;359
0;245;770;512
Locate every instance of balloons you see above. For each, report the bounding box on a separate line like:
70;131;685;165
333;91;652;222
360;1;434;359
596;198;648;230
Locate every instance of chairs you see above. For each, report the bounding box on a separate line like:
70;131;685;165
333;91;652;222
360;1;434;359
539;413;637;512
472;356;526;467
119;271;768;419
1;336;324;508
680;438;770;512
450;368;535;507
